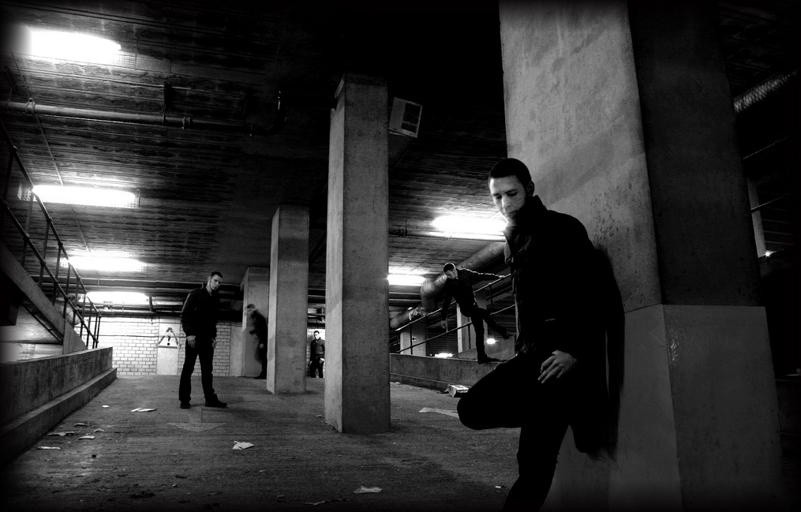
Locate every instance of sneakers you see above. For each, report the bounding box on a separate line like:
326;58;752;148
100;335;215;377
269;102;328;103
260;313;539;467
180;400;191;409
205;394;227;407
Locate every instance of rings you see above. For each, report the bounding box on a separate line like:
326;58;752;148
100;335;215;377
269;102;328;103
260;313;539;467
557;363;565;370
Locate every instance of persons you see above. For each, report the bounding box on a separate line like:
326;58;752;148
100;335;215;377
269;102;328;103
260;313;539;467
178;269;229;410
307;330;327;378
243;303;269;380
440;262;513;365
457;158;612;510
